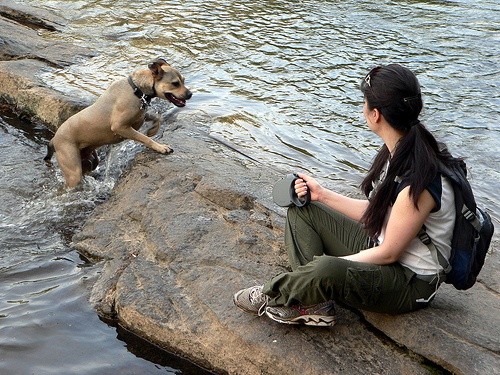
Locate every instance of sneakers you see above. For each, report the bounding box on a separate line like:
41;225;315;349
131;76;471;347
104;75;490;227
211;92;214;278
265;300;337;327
232;285;266;318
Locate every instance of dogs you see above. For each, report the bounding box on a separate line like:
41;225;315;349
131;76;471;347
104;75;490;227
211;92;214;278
41;56;194;191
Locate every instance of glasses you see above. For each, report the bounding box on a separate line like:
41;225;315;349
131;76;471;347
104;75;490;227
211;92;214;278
364;66;384;106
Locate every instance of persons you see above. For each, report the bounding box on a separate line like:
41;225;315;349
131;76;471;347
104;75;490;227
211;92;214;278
233;65;458;328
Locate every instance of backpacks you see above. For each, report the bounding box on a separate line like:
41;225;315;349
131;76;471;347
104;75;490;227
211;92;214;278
438;140;495;291
